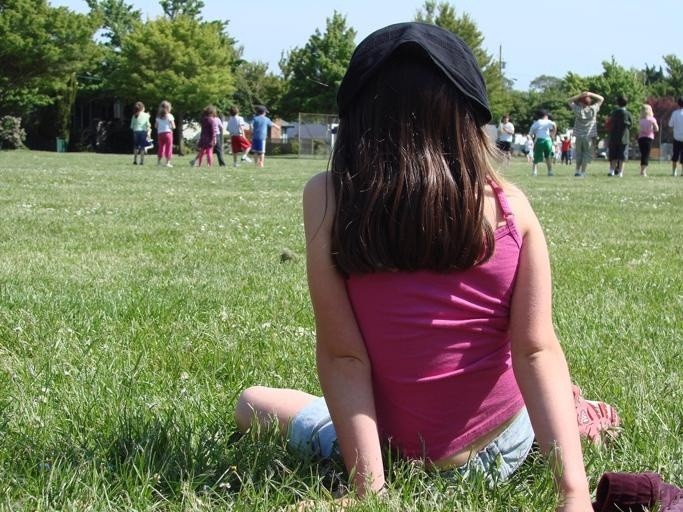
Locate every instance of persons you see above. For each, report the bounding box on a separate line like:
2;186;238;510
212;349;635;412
251;102;283;168
144;111;155;156
154;100;176;168
495;90;682;177
234;19;620;512
198;106;217;168
129;99;151;166
188;107;226;168
226;105;252;168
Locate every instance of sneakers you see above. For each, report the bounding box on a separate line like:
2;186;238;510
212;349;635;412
571;385;620;447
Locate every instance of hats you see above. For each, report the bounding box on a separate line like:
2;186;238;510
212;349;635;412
258;106;269;114
